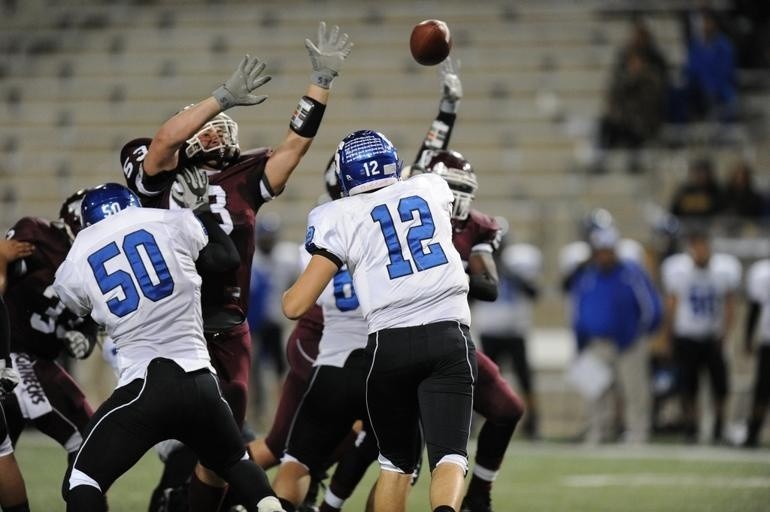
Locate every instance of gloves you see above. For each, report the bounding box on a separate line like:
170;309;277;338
304;21;354;89
211;54;272;112
439;56;462;115
171;166;211;217
63;330;90;359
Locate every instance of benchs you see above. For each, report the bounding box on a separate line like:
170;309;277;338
1;0;770;443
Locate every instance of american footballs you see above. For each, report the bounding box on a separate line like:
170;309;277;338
410;19;450;65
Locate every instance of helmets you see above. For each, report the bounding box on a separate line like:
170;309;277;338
81;182;143;227
59;190;86;238
425;149;479;221
177;103;240;167
335;129;403;198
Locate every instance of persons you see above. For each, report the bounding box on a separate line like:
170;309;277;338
582;1;770;258
556;204;770;448
2;19;545;512
